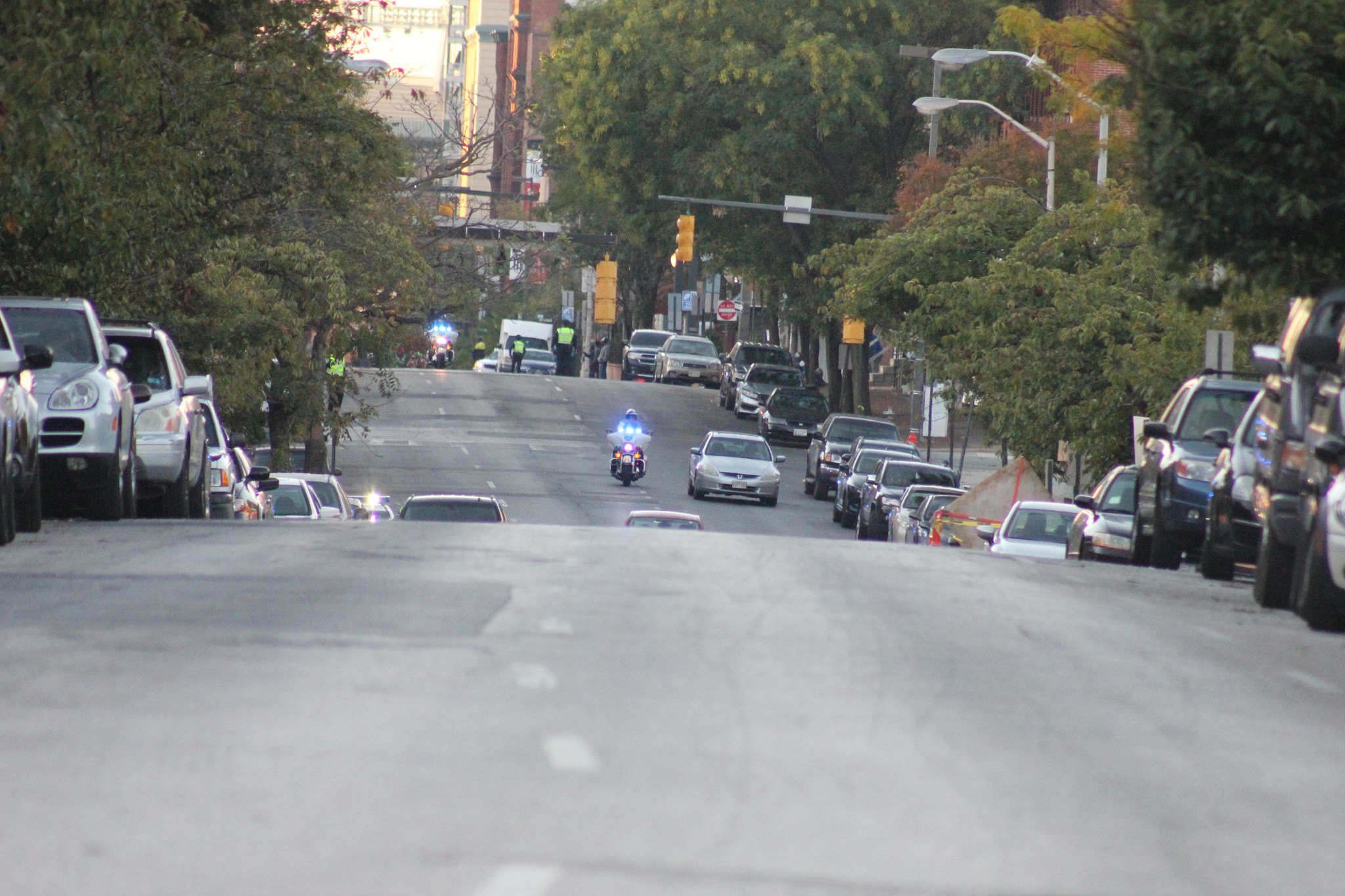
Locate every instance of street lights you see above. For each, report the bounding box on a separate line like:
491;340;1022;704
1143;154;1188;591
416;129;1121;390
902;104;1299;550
510;174;533;314
900;42;1110;211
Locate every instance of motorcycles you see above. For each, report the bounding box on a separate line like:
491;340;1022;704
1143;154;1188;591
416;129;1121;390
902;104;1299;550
605;428;653;487
424;326;459;369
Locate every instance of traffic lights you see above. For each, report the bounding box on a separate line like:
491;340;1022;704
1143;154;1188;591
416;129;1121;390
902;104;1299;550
675;215;695;262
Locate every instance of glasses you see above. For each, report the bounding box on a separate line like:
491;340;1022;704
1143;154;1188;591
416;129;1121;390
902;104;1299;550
630;415;637;419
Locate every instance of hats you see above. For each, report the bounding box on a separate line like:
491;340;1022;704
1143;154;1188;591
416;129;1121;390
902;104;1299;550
600;337;607;342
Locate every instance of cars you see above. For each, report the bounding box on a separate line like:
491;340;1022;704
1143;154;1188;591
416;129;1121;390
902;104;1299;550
654;334;726;390
0;297;393;546
688;431;786;507
393;494;507;523
978;290;1345;632
757;388;831;447
520;348;557;375
734;363;815;420
472;348;499;373
624;511;705;531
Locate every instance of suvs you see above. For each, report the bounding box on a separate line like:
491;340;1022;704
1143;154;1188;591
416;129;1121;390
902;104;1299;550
804;413;971;545
621;329;678;381
719;341;800;410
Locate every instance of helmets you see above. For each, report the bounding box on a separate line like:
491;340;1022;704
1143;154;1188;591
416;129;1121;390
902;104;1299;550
626;409;637;416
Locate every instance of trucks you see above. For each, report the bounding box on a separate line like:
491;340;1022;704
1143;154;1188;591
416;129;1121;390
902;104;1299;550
497;318;553;372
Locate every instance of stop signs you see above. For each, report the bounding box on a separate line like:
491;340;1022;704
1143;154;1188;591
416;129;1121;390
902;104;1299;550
717;300;737;321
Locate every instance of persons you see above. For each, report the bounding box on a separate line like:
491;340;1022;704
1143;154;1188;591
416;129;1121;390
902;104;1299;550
742;445;765;460
326;353;346;426
612;409;644;474
350;316;453;369
472;337;485;360
550;319;578;376
509;335;527;373
127;358;161;385
583;337;611;379
